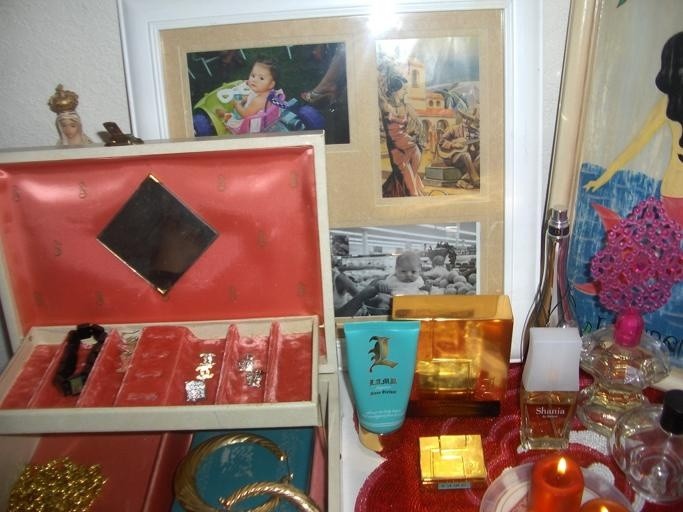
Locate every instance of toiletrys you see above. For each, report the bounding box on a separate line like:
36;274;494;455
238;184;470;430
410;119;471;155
518;326;584;451
574;312;671;440
342;321;421;453
608;390;682;504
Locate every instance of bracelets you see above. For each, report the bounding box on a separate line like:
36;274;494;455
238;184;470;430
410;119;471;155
52;323;107;397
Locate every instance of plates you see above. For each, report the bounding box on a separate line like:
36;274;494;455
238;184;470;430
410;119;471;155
478;464;632;512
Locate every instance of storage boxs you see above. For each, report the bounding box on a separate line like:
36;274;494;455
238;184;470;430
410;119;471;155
0;128;346;512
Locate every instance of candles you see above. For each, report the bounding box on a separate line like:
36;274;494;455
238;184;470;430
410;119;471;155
525;454;586;512
578;497;629;512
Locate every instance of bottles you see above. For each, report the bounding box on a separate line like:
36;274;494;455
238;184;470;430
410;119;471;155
577;305;672;438
611;386;683;504
522;209;582;362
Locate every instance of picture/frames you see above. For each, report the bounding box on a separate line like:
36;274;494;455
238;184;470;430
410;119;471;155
538;1;682;398
114;1;546;372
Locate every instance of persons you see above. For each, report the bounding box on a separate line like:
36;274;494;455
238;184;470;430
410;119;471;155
377;72;428;197
299;44;345;114
331;234;475;316
214;56;285;135
436;105;480;189
45;83;93;146
